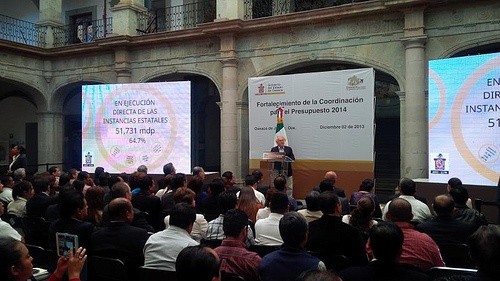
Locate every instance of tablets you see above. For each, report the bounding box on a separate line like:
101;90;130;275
56;232;78;258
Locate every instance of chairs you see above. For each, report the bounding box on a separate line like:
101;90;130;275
24;243;57;273
307;249;350;273
88;255;129;281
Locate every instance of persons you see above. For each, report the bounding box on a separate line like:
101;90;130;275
271;135;295;196
9;146;27;173
0;162;500;281
